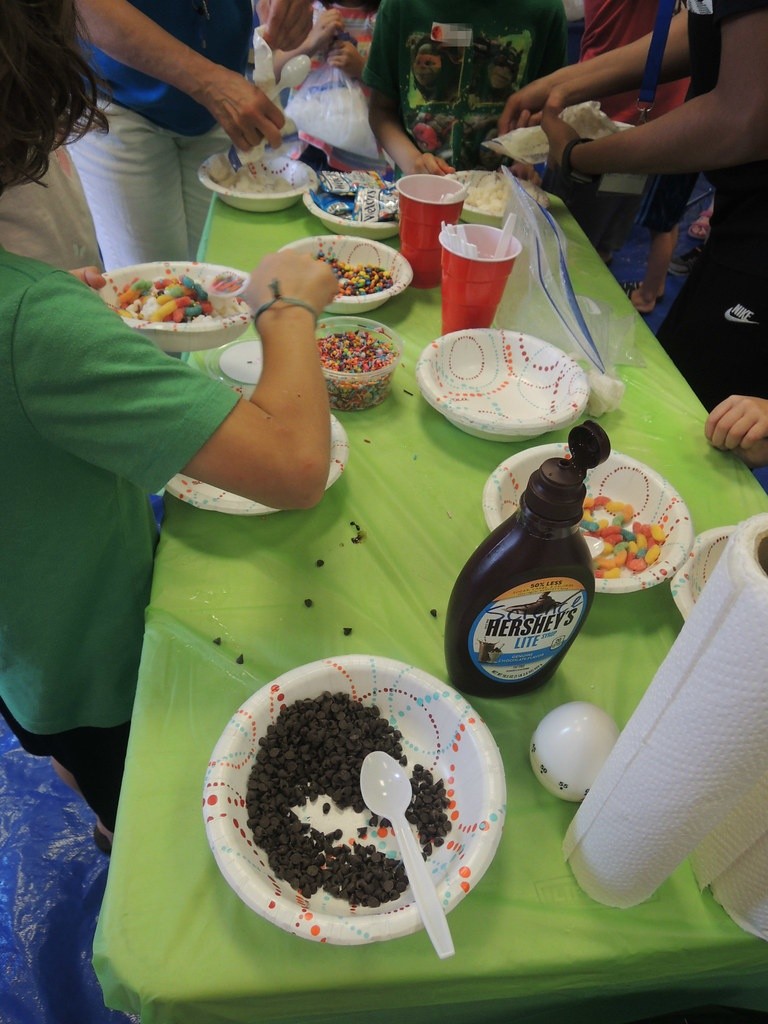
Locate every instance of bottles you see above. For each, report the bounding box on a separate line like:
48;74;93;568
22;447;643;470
445;421;611;701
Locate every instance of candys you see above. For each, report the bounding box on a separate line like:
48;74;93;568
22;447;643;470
310;169;403;224
117;273;216;325
316;252;396;295
578;495;667;577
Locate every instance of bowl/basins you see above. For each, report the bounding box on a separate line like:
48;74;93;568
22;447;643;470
442;169;551;229
163;386;349;516
314;316;403;411
201;655;507;946
483;442;694;594
670;525;738;620
197;152;317;212
93;261;252;353
302;180;399;240
416;329;591;444
276;234;414;314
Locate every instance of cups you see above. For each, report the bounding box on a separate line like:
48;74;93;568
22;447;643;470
396;174;466;289
438;224;523;336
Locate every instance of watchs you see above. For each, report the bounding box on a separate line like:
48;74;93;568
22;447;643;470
563;136;603;186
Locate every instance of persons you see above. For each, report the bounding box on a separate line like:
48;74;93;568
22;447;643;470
361;0;768;490
67;0;316;267
1;147;100;273
277;1;389;179
1;0;332;853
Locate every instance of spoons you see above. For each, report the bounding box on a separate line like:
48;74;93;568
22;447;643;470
203;269;252;296
359;751;456;960
266;53;312;101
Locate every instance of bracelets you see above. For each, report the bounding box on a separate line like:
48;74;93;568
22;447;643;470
254;280;319;329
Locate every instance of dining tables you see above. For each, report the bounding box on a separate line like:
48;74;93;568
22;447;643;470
93;176;767;1024
